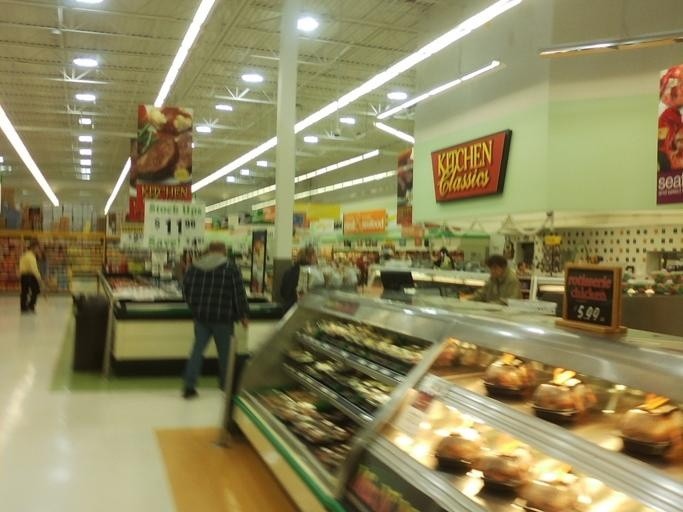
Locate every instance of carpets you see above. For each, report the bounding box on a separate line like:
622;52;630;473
154;425;300;512
51;312;222;391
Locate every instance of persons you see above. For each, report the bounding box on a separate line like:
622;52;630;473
433;247;454;271
280;241;318;314
517;262;531;290
459;254;522;305
252;237;264;294
180;241;251;398
36;246;48;300
17;243;41;313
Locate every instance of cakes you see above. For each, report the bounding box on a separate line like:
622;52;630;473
434;353;683;512
433;339;479;367
